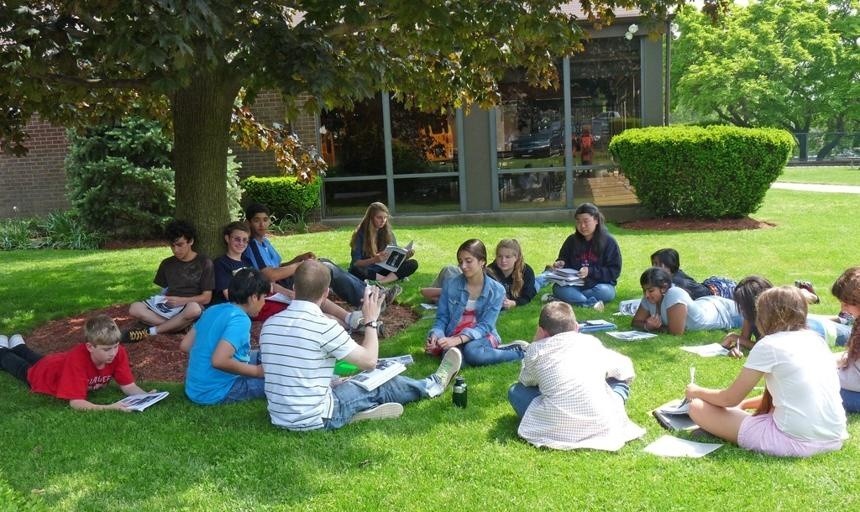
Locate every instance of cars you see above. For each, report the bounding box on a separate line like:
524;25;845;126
512;129;561;157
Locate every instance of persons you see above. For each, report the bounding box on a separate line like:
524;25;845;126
497;164;560;200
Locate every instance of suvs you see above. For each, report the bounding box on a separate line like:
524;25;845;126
593;112;622;120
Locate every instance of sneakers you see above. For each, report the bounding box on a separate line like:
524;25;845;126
541;292;561;302
348;311;362;327
356;320;386;338
364;278;381;287
120;326;151;345
436;347;463;389
800;281;819;305
398;277;409;283
352;401;404;422
0;335;9;349
9;333;26;349
497;340;529;354
839;309;855;325
592;300;604;312
384;285;400;306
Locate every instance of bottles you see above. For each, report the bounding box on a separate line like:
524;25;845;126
453;378;467;408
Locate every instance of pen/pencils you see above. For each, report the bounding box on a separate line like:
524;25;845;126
358;292;373;310
736;335;740;354
584;324;604;326
